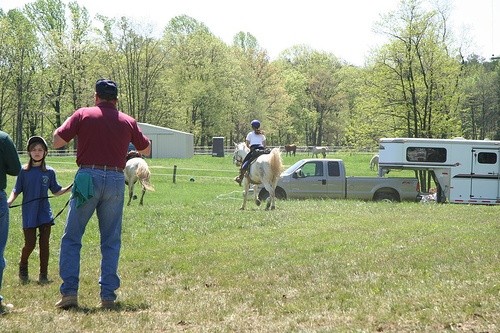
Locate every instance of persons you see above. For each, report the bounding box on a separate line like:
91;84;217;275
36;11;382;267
235;120;267;182
53;78;152;311
0;130;21;315
8;135;73;285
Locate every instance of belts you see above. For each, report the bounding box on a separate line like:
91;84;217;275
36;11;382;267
80;164;124;171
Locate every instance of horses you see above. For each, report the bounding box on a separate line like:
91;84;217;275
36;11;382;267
285;145;296;156
231;140;282;211
312;147;326;158
123;157;155;206
370;155;379;172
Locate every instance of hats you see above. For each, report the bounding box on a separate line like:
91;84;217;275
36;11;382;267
96;78;117;94
27;136;49;151
251;120;260;128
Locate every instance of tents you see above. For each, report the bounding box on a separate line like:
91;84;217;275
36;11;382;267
136;122;194;159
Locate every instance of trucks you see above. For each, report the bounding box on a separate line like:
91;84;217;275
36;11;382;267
377;138;498;205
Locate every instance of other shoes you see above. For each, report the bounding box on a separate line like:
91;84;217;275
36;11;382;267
55;296;78;309
101;299;114;305
39;277;48;285
19;260;28;279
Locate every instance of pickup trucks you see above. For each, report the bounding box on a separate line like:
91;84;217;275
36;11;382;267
254;158;421;203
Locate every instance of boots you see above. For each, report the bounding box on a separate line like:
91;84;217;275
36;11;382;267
235;169;246;182
